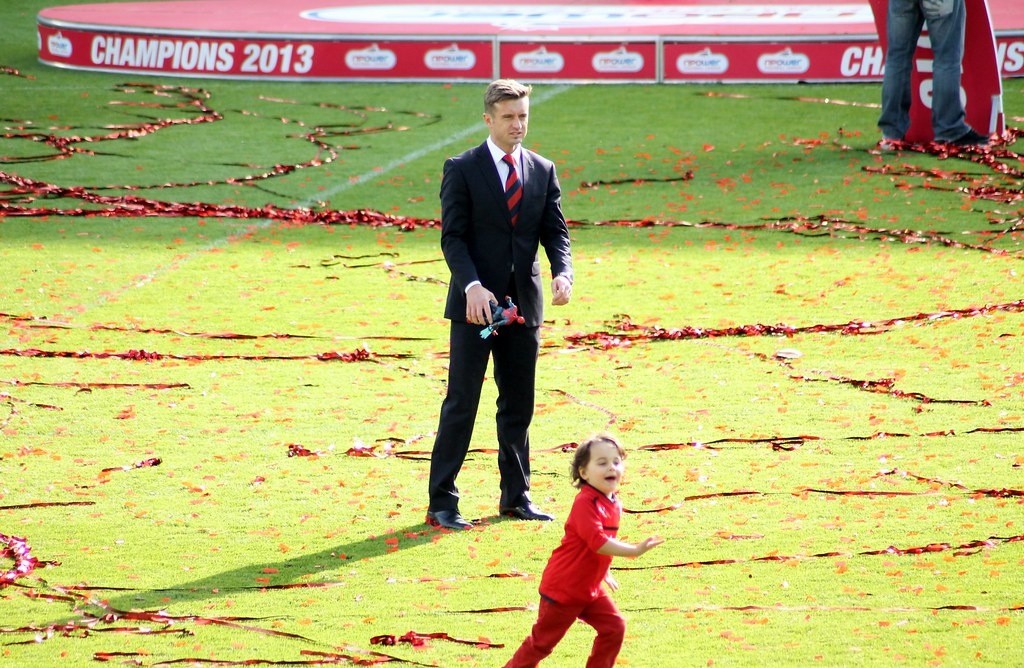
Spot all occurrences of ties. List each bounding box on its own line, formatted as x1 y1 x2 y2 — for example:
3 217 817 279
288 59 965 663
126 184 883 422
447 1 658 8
501 153 522 226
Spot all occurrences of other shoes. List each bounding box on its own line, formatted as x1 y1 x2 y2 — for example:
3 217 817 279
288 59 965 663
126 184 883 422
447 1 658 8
937 129 990 146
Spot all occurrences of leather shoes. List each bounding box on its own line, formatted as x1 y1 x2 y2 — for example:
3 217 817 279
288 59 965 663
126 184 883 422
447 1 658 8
426 509 473 529
500 501 554 520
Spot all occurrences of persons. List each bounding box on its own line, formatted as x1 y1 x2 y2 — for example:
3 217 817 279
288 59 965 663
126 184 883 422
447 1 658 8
425 77 573 528
877 1 993 148
500 437 664 668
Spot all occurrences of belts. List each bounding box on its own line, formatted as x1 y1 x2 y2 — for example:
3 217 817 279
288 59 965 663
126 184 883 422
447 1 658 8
506 264 515 272
